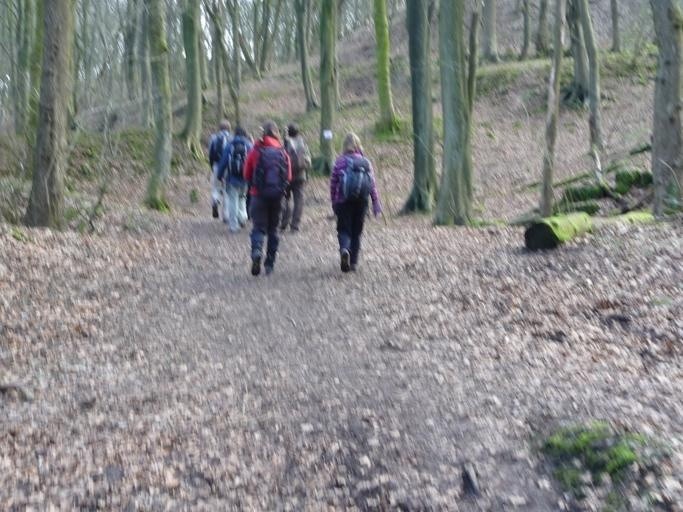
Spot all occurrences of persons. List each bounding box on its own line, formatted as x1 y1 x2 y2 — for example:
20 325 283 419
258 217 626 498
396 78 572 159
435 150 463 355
241 119 294 275
279 121 313 232
217 124 253 234
329 129 382 273
208 119 235 223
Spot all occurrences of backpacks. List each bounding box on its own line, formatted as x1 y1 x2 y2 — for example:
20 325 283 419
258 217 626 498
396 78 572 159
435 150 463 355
227 141 248 177
341 154 371 204
253 145 290 199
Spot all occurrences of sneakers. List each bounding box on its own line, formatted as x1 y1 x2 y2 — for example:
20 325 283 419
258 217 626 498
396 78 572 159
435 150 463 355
213 204 218 217
341 249 350 272
252 255 261 276
